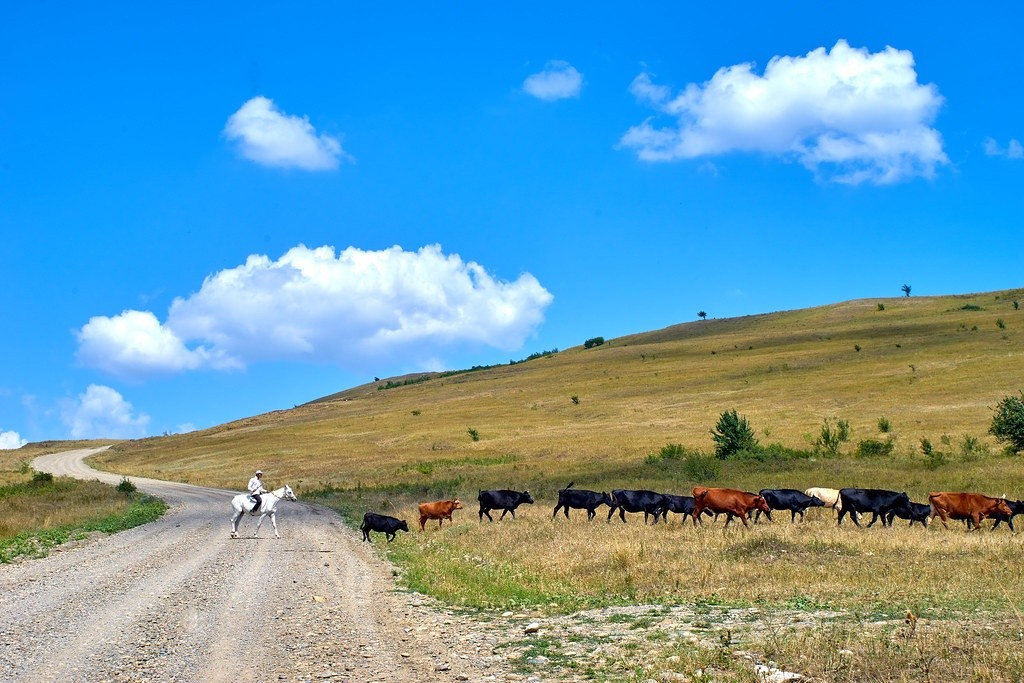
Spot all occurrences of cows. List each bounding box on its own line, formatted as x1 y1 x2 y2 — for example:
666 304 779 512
360 513 409 544
553 482 613 521
606 486 1024 532
478 489 534 522
418 498 463 531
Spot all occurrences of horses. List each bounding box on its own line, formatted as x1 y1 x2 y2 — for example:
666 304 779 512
231 484 298 539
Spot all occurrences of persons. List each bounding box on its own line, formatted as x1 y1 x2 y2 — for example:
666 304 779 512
248 470 268 516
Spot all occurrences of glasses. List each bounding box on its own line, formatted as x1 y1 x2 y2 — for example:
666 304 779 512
261 474 262 475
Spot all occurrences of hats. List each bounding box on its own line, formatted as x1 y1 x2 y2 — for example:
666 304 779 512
256 470 263 473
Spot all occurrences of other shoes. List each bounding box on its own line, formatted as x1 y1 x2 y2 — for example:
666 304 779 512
249 512 254 516
